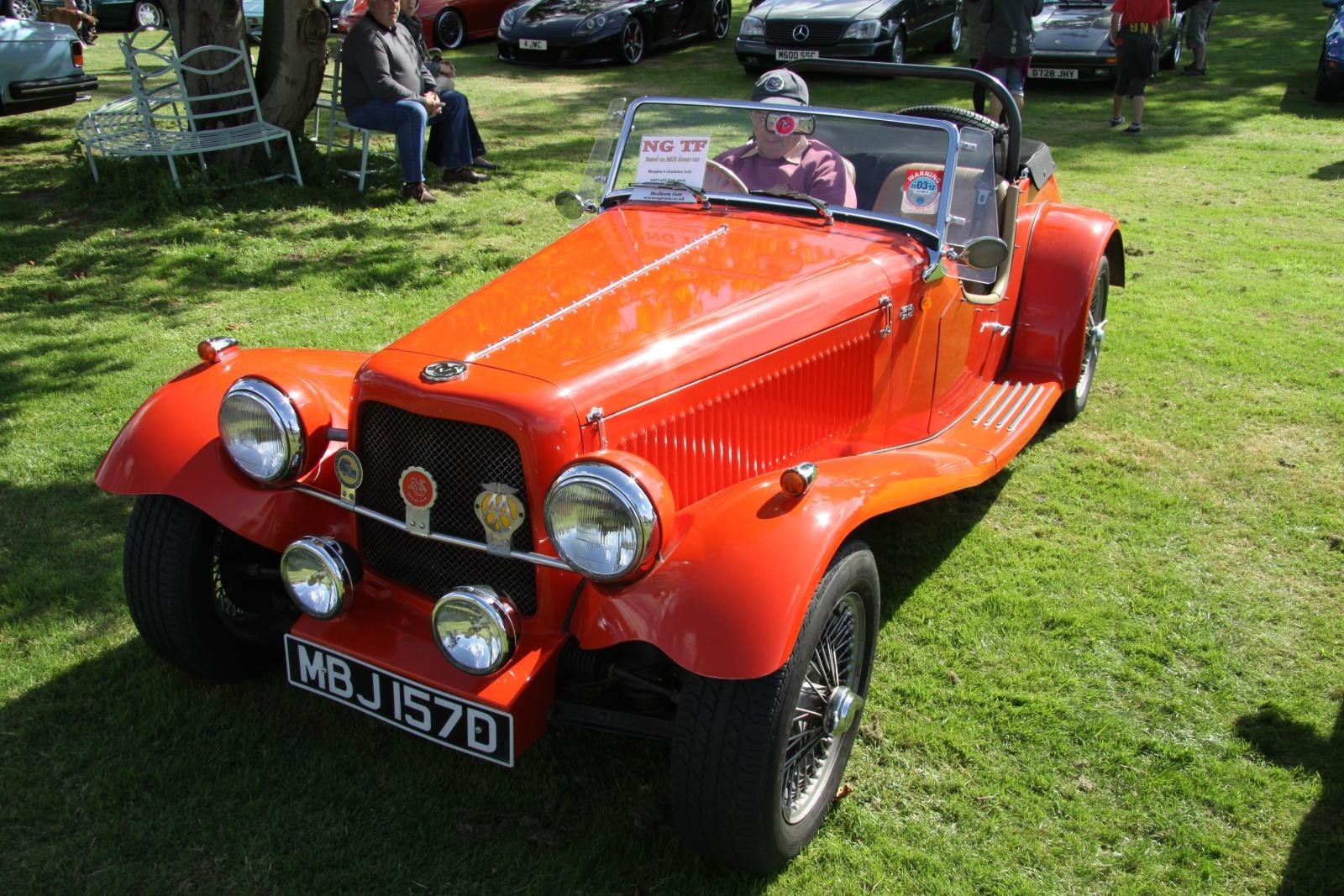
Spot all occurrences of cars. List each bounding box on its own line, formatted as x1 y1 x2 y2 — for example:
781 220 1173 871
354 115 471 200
1318 0 1344 83
0 0 168 33
95 55 1131 880
241 0 353 44
335 0 510 62
0 18 98 116
493 0 732 64
732 0 966 79
1021 0 1186 90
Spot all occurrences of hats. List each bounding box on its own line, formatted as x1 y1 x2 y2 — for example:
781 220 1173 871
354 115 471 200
751 67 809 106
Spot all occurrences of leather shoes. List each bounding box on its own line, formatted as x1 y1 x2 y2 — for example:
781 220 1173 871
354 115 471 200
402 182 438 204
442 166 491 184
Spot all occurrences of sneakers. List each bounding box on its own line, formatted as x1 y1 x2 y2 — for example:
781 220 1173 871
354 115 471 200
1110 115 1126 129
1122 126 1142 136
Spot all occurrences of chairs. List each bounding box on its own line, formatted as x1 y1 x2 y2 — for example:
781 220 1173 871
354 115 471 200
871 163 1009 294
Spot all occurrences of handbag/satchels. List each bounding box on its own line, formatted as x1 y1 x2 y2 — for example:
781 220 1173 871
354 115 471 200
420 48 455 94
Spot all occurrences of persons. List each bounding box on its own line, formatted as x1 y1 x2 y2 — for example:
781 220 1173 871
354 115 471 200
1109 0 1172 136
1170 0 1221 75
69 0 99 46
959 0 1044 125
397 0 501 171
340 0 491 202
702 68 857 209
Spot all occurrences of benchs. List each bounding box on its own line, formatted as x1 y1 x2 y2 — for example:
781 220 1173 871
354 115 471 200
60 17 403 195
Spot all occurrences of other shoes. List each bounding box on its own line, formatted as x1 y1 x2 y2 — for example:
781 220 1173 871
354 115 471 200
1179 61 1206 77
472 156 500 170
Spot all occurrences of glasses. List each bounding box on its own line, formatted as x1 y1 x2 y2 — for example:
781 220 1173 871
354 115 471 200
748 111 766 122
